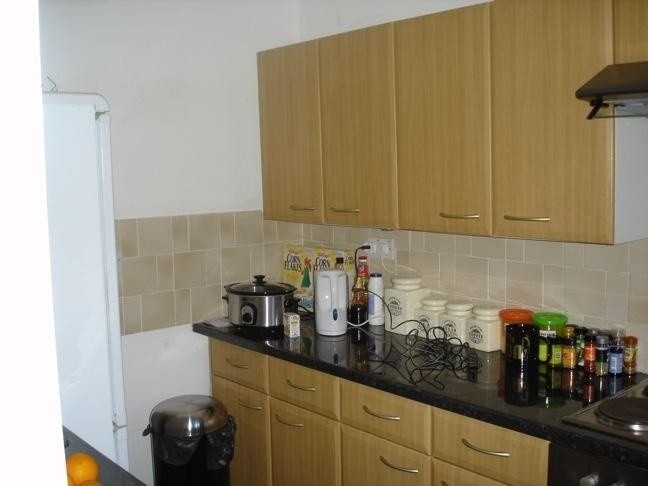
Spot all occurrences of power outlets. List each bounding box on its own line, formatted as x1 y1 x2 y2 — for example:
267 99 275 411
364 238 395 257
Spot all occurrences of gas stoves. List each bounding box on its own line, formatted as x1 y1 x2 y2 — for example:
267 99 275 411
561 378 648 446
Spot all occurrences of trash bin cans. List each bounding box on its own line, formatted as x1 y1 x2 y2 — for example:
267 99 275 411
142 394 238 486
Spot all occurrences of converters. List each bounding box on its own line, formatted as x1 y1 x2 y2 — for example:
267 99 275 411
467 348 478 370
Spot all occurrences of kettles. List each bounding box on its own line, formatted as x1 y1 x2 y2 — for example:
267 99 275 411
313 269 348 337
316 335 347 368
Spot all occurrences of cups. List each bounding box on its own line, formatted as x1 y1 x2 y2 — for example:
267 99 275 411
503 370 552 407
505 323 553 369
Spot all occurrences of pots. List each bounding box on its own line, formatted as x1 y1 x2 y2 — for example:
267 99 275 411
221 275 301 340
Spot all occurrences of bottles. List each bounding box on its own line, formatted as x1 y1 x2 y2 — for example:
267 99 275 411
538 367 563 402
351 256 370 310
562 327 638 376
350 346 367 377
367 273 385 325
369 326 386 372
351 289 366 346
532 312 568 367
500 310 534 354
562 368 634 409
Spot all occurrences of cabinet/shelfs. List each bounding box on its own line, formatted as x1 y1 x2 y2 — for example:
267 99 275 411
396 0 615 261
256 19 399 232
614 1 648 67
208 337 342 485
341 378 550 485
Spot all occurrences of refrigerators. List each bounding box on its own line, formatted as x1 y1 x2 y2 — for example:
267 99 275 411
43 92 130 472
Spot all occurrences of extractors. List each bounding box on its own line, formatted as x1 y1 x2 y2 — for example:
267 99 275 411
575 61 648 121
